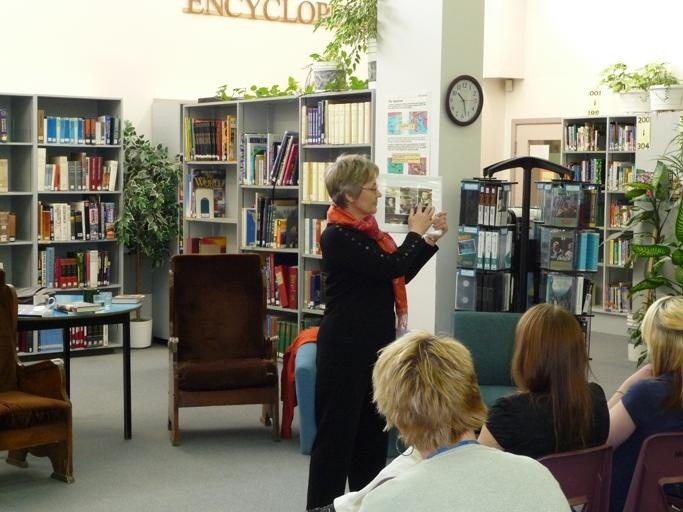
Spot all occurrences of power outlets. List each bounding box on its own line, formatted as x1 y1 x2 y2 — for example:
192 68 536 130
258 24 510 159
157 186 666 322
560 110 683 315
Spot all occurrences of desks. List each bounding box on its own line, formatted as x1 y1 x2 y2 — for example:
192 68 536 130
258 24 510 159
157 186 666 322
17 296 142 442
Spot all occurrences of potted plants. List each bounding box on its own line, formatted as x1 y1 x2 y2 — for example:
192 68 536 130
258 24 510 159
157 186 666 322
301 0 378 95
602 58 647 112
117 121 180 352
596 159 682 363
646 60 682 111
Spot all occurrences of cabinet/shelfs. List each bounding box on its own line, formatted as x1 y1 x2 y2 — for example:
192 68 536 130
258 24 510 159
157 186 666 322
0 93 32 290
182 96 297 352
299 91 374 313
34 100 125 353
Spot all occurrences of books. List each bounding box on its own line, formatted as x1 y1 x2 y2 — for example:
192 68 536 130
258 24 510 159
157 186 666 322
240 131 299 185
304 217 327 256
191 236 227 254
448 113 639 315
185 168 226 218
1 102 144 353
301 100 372 146
241 192 298 248
262 253 298 309
301 315 321 331
184 114 237 162
304 269 327 309
264 313 298 358
302 161 337 202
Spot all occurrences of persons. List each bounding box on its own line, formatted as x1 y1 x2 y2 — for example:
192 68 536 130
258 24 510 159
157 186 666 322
302 152 449 510
333 329 574 509
458 303 613 459
605 292 682 511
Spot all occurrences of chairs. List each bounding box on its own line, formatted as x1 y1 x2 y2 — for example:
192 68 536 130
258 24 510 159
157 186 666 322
294 326 415 459
164 251 281 445
535 443 621 511
0 268 75 483
626 431 682 512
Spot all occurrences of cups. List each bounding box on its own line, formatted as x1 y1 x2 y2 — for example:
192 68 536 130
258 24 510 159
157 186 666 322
92 295 105 313
32 294 55 315
99 291 112 310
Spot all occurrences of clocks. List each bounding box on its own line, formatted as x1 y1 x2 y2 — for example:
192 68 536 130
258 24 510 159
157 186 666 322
443 74 484 126
